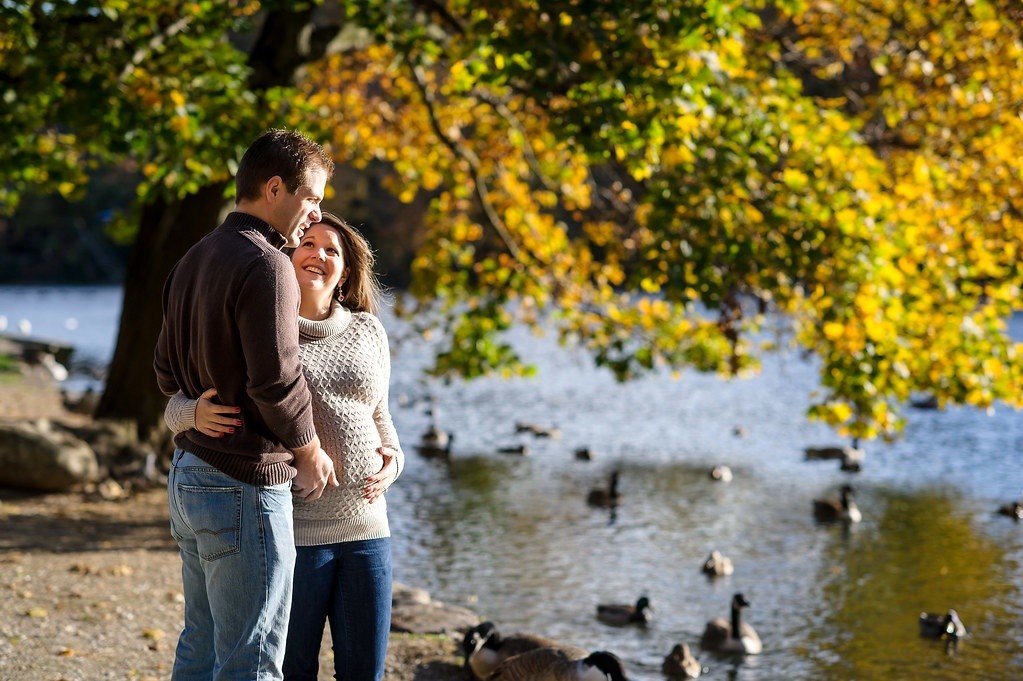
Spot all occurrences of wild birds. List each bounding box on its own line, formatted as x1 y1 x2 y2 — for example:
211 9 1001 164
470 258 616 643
660 643 702 680
998 500 1023 521
812 482 863 528
702 549 735 578
906 390 941 413
700 591 764 656
496 420 563 455
597 593 656 626
587 466 623 508
801 436 866 472
916 605 968 643
709 462 733 483
409 407 455 466
463 620 627 681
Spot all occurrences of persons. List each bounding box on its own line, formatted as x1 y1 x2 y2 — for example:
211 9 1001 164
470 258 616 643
164 212 405 681
155 127 341 681
